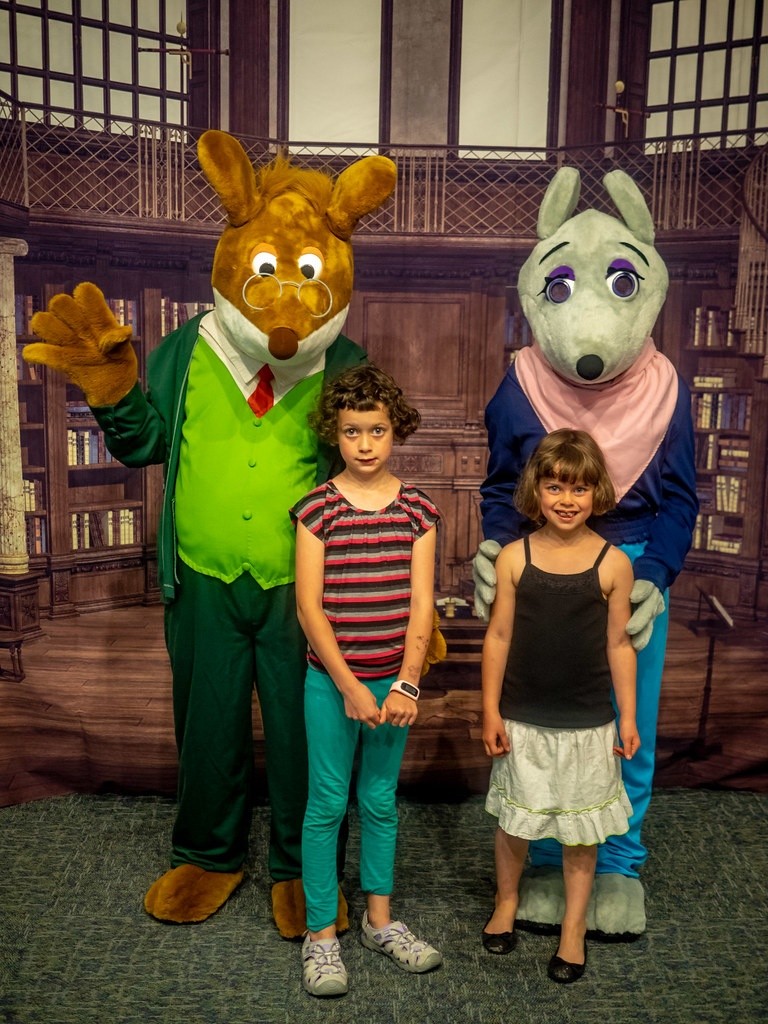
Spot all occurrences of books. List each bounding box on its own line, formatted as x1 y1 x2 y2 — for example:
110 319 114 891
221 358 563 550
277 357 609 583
684 301 750 553
14 296 217 557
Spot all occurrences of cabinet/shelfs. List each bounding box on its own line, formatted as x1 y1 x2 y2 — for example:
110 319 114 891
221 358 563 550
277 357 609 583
15 282 216 620
664 278 768 622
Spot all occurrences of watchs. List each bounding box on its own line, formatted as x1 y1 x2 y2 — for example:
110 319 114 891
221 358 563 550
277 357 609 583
390 680 420 703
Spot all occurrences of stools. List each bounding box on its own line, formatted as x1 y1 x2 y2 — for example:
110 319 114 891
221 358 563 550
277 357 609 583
0 630 26 683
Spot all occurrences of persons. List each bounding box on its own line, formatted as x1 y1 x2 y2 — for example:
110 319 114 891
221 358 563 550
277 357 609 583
481 429 640 983
287 367 446 996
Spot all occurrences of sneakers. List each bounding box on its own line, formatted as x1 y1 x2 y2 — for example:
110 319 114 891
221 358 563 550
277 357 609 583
300 931 349 995
361 909 443 972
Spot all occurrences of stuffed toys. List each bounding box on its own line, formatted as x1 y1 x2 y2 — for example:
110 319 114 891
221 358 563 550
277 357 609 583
472 165 699 938
21 129 444 935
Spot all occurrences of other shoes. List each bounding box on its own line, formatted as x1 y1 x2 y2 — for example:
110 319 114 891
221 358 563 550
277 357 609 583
547 939 588 985
482 906 518 955
